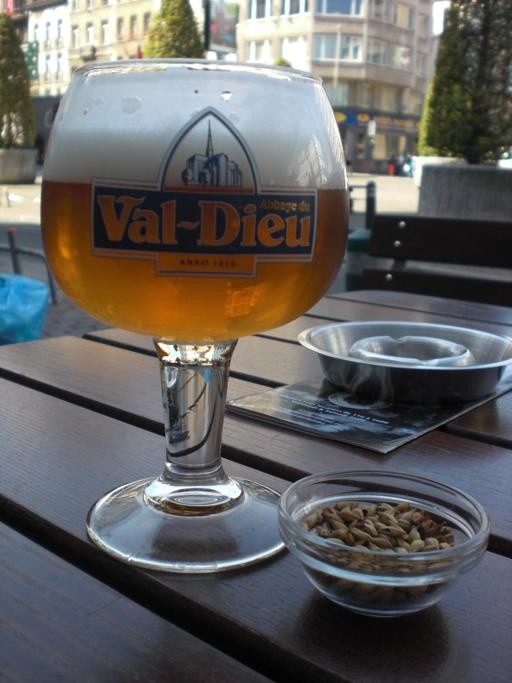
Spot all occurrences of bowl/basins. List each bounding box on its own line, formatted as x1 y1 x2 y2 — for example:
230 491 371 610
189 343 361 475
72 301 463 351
276 468 493 621
297 318 512 406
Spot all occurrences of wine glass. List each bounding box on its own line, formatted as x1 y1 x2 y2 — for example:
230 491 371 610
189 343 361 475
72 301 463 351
37 56 353 577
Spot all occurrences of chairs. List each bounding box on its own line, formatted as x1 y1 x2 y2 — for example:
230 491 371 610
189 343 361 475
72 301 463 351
364 212 511 306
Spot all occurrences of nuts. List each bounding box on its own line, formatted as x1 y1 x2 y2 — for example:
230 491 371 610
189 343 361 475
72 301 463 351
302 498 455 609
